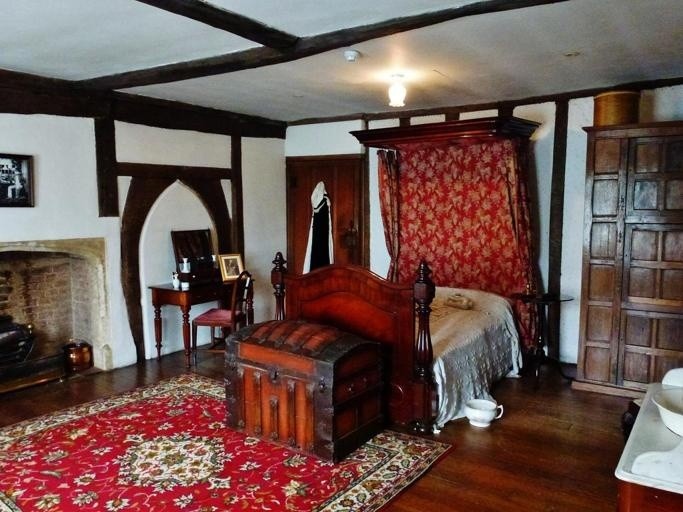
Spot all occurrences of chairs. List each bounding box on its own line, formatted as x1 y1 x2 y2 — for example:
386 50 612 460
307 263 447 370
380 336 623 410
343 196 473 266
190 269 251 366
662 365 683 389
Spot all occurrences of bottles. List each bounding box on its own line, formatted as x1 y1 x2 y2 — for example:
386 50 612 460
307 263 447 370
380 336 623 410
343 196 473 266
180 257 190 288
171 273 179 288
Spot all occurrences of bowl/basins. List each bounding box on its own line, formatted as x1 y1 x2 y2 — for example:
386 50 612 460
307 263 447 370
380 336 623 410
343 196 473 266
650 385 683 437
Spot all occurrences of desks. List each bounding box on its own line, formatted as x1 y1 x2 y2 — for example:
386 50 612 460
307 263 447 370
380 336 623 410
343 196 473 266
611 382 682 511
512 289 576 391
147 277 254 371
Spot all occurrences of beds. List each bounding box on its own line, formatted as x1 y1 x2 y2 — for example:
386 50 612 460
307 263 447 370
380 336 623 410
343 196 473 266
269 113 540 432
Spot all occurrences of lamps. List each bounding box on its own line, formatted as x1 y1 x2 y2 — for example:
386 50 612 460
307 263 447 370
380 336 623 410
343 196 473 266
385 74 406 107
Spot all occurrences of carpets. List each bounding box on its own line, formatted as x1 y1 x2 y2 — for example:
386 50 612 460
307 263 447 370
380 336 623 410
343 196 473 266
1 370 453 512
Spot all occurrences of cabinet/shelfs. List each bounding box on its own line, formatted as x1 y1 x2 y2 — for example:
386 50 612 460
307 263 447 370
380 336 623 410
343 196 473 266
571 119 683 397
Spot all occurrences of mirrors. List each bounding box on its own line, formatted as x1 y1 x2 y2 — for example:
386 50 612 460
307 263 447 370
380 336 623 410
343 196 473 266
170 228 220 285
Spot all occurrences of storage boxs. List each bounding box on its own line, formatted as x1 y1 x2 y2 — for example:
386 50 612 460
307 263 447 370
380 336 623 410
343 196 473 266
222 317 394 464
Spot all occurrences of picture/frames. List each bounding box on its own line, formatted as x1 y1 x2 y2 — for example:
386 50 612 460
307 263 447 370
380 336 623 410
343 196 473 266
0 151 35 209
217 254 244 282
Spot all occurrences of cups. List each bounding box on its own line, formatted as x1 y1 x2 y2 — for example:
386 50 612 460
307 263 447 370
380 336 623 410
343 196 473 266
61 342 90 372
464 398 503 427
